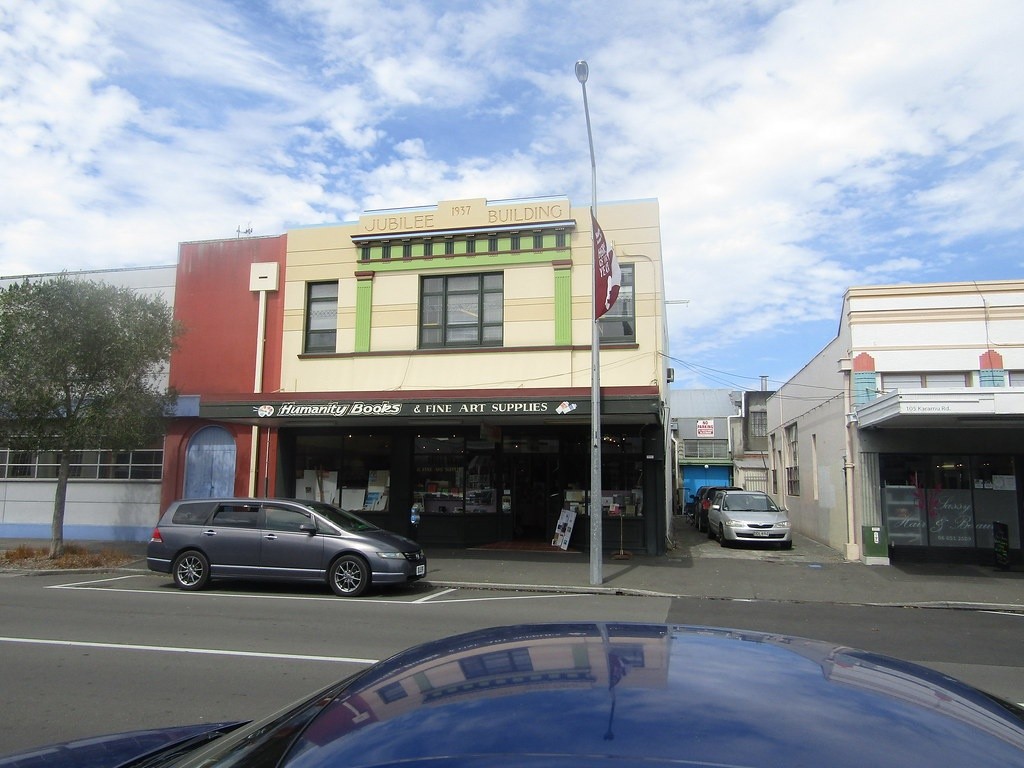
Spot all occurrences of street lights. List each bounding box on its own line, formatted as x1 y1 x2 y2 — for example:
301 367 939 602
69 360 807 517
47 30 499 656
574 57 605 585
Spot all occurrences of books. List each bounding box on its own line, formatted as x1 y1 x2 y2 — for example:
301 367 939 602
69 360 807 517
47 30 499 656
428 484 458 498
566 491 591 515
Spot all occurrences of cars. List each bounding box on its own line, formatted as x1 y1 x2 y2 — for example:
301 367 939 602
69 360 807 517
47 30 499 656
689 486 744 532
145 498 428 597
707 491 793 551
1 620 1024 768
